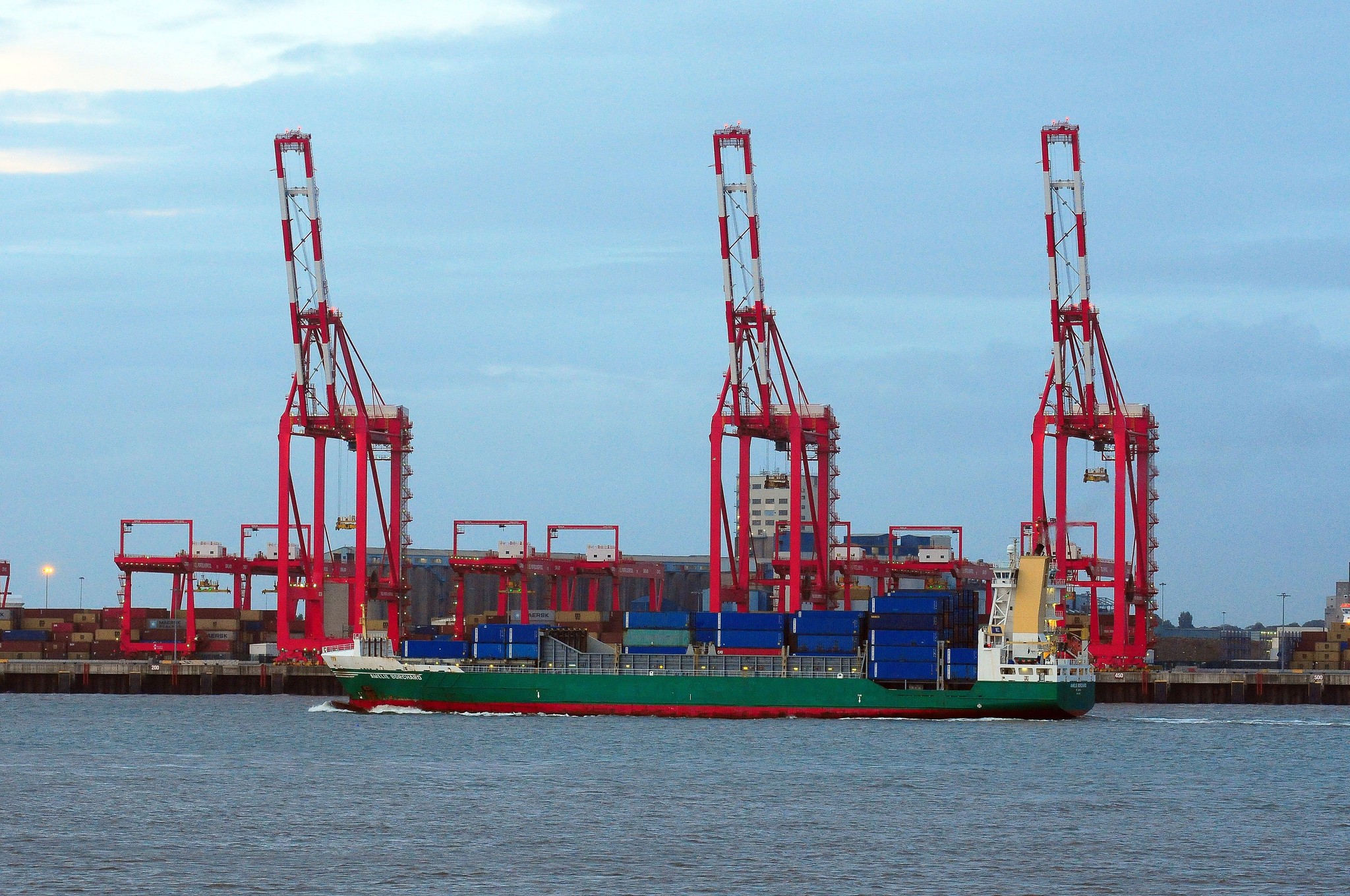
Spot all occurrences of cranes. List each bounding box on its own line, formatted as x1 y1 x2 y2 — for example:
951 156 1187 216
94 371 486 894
274 132 412 662
1029 117 1159 671
710 128 839 657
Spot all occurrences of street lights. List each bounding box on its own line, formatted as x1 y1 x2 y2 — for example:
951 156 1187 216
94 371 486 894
1159 583 1166 619
44 568 52 609
1276 593 1291 670
1222 612 1226 630
79 577 84 609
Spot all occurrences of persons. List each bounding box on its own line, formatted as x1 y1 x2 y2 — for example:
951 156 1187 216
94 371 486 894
1162 659 1176 671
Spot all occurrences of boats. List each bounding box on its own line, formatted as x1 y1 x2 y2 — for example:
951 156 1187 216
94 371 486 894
321 538 1095 719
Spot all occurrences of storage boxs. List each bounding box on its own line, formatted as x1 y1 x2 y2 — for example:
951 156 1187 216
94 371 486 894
1065 613 1271 662
366 610 623 659
624 590 978 680
0 607 306 660
1289 622 1350 670
